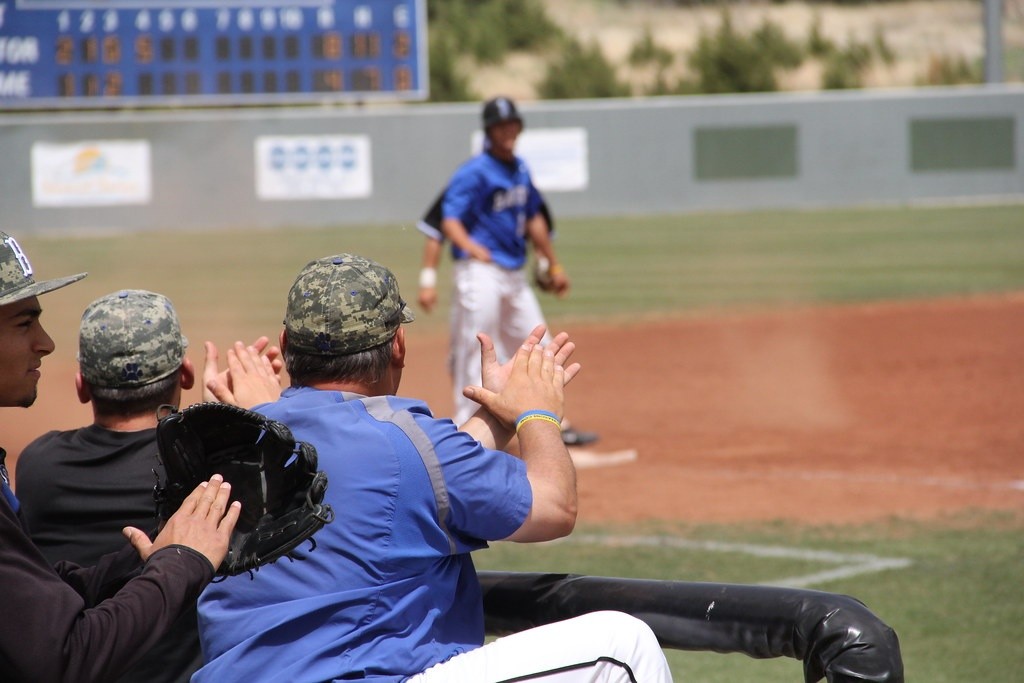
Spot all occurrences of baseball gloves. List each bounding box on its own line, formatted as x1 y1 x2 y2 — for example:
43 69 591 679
151 401 336 576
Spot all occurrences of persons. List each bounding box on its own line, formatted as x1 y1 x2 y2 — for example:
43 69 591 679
0 228 242 683
190 253 673 683
15 287 283 568
414 95 599 446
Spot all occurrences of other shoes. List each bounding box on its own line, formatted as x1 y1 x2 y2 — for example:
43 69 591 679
561 426 602 445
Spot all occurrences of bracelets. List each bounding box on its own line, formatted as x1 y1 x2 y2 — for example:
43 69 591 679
418 267 437 288
514 409 562 439
549 264 563 276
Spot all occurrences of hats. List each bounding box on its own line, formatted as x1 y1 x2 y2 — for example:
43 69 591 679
76 289 189 390
283 253 415 358
0 230 88 307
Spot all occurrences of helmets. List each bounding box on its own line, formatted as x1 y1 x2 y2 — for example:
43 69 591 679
482 97 523 138
